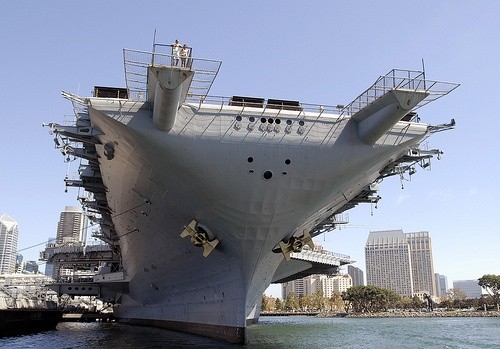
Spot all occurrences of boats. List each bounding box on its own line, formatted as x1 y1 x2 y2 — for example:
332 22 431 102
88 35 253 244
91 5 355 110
0 305 64 336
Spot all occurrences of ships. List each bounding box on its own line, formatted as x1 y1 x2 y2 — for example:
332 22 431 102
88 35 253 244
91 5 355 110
41 28 460 343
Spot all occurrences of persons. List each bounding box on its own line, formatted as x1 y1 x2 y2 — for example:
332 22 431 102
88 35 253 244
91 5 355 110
172 39 182 66
180 44 189 67
